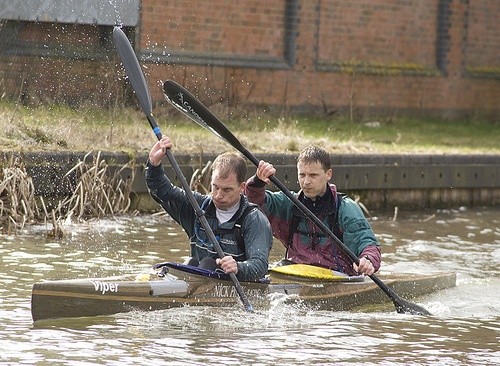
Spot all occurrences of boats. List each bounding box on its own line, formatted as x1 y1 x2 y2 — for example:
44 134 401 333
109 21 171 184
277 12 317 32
31 270 459 325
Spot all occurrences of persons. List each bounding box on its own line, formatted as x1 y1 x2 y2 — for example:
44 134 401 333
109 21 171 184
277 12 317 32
245 144 382 277
145 135 274 282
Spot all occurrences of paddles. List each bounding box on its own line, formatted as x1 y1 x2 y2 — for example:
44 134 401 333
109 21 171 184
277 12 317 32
111 26 253 314
163 79 434 320
